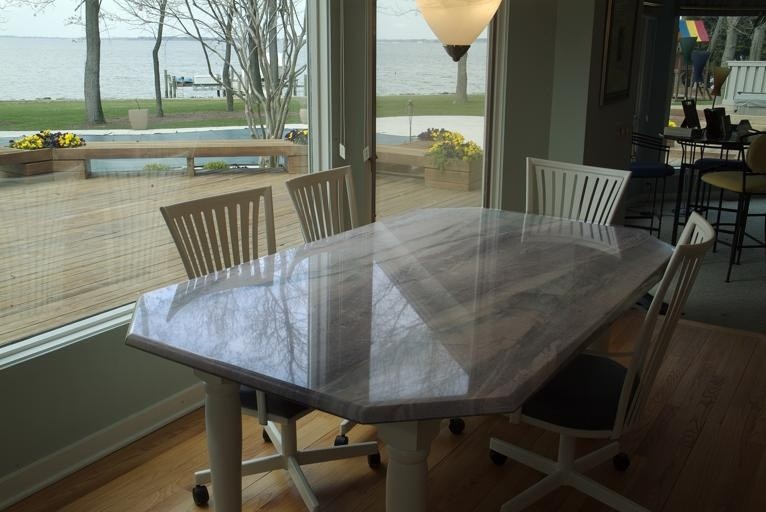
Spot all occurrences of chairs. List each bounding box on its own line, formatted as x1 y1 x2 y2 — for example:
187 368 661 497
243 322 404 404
630 100 766 283
524 158 634 225
285 163 466 449
159 186 382 511
487 211 716 509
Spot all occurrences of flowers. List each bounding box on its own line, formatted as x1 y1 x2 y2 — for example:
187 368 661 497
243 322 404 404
285 129 309 145
424 138 486 172
416 128 464 144
7 129 86 150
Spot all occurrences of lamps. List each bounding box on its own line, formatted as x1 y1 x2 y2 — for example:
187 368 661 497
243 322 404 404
417 0 503 64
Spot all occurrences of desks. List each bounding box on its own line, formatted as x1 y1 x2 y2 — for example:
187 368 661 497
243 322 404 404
126 207 676 512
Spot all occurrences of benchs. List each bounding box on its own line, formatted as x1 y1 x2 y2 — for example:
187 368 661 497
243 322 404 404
85 138 289 176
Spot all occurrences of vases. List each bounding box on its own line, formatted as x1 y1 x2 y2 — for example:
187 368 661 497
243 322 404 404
0 146 91 182
424 154 482 192
281 149 310 173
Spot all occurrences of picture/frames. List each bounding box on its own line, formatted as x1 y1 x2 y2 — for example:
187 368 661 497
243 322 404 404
600 1 640 105
